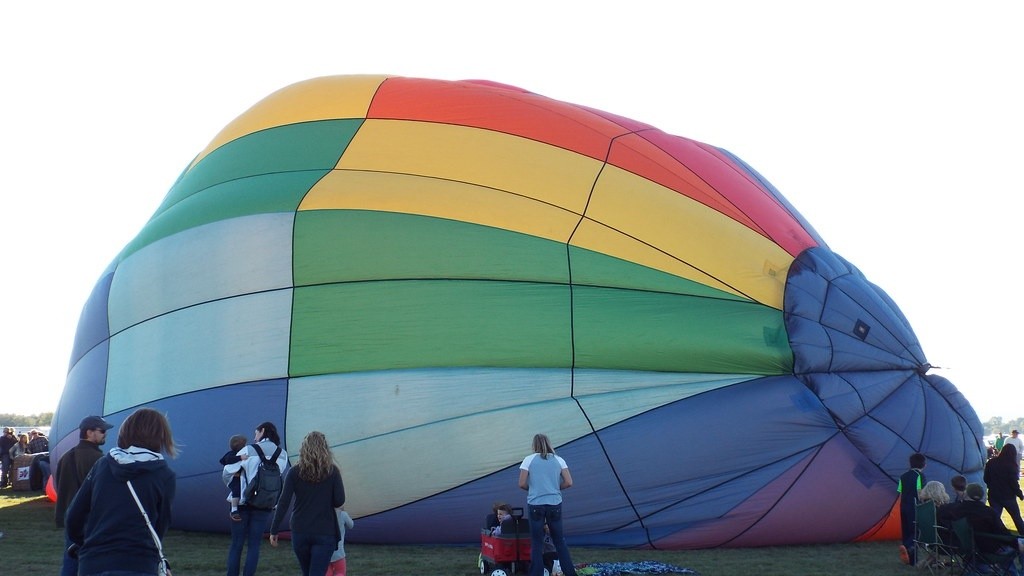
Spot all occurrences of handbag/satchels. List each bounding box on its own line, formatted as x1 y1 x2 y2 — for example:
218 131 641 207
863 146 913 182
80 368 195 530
67 543 79 559
157 557 171 576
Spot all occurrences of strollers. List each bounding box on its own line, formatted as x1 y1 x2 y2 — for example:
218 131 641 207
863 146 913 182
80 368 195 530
480 506 551 576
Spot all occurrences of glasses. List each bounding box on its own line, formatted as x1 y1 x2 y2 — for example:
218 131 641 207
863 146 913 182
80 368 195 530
93 428 107 435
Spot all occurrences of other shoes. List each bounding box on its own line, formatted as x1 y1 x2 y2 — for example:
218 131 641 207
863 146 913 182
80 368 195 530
230 510 242 522
899 545 909 564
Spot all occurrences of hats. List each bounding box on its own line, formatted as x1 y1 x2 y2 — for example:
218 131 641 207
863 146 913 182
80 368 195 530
79 415 114 431
1012 430 1020 434
967 483 985 501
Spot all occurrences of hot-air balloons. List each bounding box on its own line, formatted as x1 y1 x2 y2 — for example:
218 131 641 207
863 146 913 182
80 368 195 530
8 74 989 551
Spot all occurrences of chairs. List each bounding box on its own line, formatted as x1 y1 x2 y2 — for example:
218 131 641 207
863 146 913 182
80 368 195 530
911 496 969 576
948 517 1022 576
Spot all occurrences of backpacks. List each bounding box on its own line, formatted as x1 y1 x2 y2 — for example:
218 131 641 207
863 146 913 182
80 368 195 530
242 444 284 514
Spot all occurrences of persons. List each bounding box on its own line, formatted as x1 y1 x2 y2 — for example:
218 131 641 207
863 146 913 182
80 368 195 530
66 409 186 576
486 433 579 576
219 422 354 576
988 430 1024 479
984 443 1024 536
896 453 1024 576
0 428 50 494
55 416 114 576
